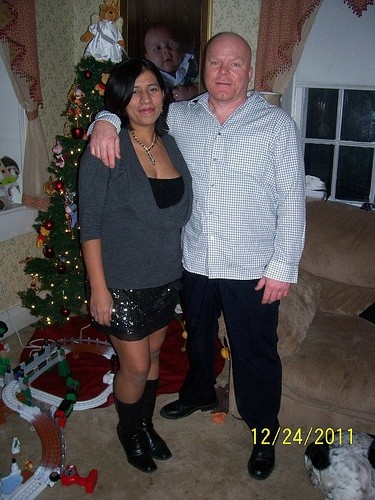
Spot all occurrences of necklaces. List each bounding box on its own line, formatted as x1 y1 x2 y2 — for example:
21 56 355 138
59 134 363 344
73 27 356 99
131 130 157 165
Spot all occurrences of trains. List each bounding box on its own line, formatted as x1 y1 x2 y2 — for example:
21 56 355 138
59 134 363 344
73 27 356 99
54 378 79 420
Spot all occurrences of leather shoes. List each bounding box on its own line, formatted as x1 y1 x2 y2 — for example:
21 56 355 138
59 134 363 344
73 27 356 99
160 398 219 419
248 425 280 480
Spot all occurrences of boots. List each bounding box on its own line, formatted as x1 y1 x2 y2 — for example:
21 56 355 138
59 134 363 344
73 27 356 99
141 377 172 459
112 391 158 473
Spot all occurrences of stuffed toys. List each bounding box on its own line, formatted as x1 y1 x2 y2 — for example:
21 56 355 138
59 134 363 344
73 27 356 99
80 2 125 63
65 202 78 228
52 144 68 168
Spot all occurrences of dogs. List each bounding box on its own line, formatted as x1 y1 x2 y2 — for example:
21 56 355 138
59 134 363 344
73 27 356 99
305 429 375 500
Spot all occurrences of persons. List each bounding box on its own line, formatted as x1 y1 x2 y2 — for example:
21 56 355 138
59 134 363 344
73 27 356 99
79 58 192 473
143 22 199 102
87 31 306 480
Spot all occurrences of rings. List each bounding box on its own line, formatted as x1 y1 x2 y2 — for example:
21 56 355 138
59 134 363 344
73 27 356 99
176 94 177 97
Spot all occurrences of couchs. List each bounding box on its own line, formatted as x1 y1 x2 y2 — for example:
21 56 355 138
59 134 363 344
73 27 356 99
215 195 375 438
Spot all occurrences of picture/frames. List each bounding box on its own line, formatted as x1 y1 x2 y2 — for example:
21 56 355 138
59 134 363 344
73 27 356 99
120 0 213 103
